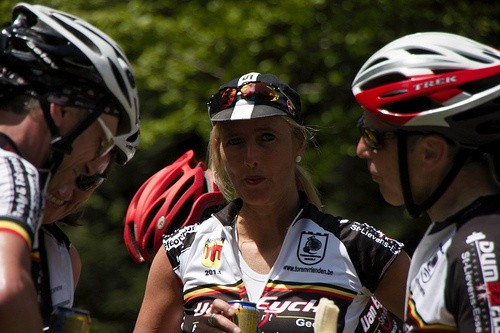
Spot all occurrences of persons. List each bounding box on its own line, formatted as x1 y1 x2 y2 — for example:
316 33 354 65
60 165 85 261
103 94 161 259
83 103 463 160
0 3 140 332
351 32 500 333
132 73 412 333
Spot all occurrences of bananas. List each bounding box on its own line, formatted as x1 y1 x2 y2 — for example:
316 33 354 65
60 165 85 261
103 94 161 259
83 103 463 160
312 297 340 333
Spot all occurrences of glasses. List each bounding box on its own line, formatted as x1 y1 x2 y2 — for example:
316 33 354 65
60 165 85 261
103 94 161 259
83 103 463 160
207 81 301 126
72 168 105 192
356 114 457 150
89 109 120 162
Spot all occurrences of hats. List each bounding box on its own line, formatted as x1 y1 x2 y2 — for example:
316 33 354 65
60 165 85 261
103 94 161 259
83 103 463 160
208 71 301 121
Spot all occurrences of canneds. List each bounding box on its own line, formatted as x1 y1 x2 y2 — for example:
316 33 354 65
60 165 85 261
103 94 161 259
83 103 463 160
47 304 92 333
223 300 257 333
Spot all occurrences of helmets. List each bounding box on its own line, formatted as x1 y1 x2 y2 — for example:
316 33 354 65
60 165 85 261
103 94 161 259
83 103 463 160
0 2 140 166
351 31 500 151
123 149 229 264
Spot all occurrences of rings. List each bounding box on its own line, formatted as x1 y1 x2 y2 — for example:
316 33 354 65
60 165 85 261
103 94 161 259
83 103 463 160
208 313 216 328
